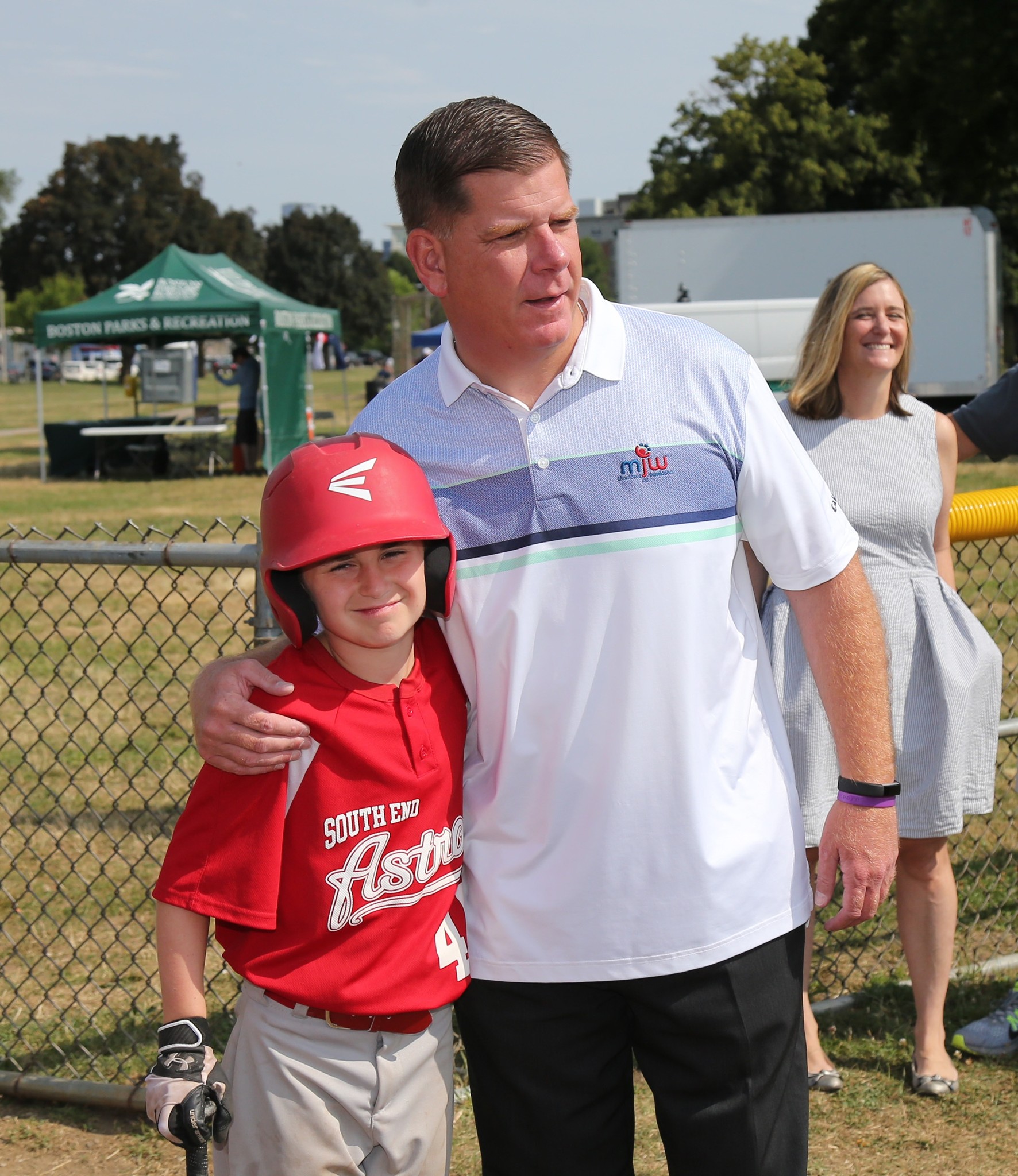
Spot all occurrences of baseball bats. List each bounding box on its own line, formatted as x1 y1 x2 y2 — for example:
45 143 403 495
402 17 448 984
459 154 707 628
183 1100 217 1176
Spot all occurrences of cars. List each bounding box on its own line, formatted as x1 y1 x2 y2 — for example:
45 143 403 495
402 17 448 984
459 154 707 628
9 342 125 386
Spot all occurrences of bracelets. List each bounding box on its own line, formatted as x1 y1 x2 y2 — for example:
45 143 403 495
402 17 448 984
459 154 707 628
837 776 901 809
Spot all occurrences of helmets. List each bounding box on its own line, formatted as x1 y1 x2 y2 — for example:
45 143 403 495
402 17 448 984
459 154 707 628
259 432 456 650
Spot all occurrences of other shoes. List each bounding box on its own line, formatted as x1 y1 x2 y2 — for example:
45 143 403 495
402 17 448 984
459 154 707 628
807 1049 846 1091
910 1049 960 1096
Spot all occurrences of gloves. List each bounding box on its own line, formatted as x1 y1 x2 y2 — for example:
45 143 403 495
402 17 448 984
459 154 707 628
147 1017 232 1152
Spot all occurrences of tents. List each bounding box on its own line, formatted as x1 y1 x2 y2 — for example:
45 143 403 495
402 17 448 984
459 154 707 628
34 243 350 484
411 320 448 367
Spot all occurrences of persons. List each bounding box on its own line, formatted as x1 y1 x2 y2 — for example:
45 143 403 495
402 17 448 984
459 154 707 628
189 99 900 1176
420 346 433 360
211 345 260 472
945 363 1018 464
17 345 60 386
141 433 469 1176
674 282 691 303
742 259 1003 1094
374 355 395 396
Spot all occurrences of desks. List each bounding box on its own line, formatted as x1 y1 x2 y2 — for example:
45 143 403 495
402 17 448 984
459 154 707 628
44 413 186 479
82 424 229 479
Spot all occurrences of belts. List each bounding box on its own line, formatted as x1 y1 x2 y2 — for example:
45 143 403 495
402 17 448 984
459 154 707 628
264 990 433 1034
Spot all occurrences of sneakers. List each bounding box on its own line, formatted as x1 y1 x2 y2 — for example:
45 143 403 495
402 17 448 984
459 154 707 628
949 992 1018 1055
